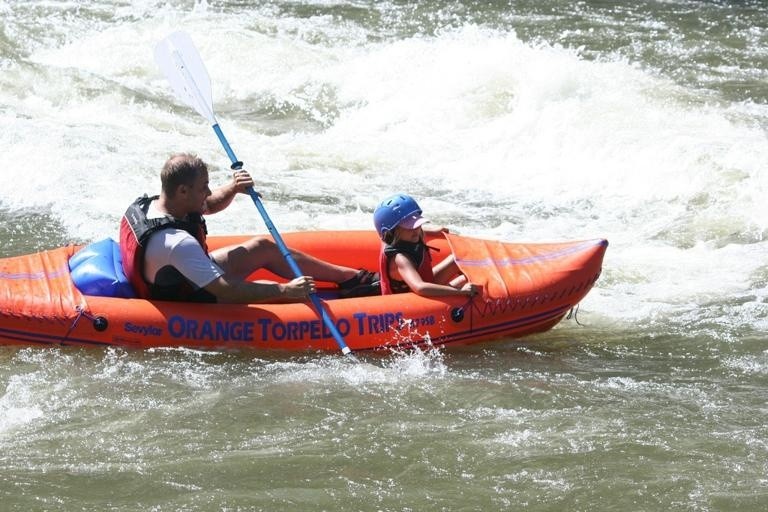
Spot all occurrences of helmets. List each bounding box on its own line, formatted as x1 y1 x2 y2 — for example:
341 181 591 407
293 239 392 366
373 194 422 240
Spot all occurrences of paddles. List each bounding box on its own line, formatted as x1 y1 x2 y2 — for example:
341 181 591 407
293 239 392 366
151 30 360 364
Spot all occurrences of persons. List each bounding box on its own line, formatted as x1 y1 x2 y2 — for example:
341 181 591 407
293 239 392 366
372 193 479 298
119 153 381 303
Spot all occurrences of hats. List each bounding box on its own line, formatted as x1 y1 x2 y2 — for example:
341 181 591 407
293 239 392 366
399 213 430 229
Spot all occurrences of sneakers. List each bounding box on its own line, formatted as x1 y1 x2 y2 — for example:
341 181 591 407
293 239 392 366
338 268 380 297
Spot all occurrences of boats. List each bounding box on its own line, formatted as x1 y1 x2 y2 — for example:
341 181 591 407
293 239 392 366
0 226 612 358
